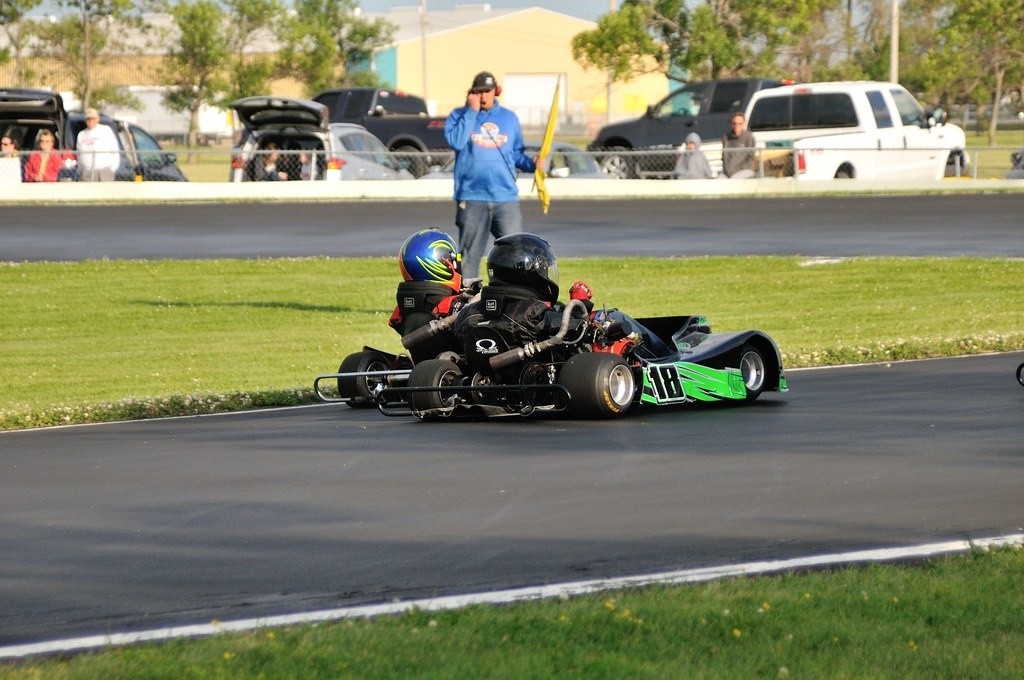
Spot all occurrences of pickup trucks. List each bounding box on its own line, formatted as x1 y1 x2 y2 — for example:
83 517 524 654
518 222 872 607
311 88 456 179
673 80 971 181
585 78 805 180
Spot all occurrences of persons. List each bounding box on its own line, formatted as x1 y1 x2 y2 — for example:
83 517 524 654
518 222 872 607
0 128 76 182
689 94 702 115
388 228 483 335
717 113 756 179
674 132 713 179
253 142 322 181
454 233 676 361
444 71 547 279
76 107 120 182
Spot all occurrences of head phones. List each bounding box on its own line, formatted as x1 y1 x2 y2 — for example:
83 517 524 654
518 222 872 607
495 83 501 97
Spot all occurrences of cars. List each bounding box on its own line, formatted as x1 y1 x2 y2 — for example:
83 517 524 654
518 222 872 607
417 140 615 179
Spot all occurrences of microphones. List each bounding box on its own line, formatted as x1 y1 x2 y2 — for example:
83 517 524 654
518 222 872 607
480 102 486 105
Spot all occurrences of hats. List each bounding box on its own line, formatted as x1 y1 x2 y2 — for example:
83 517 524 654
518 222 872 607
84 108 98 119
471 71 496 92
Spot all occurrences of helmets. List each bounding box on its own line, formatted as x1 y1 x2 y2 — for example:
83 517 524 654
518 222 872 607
487 232 560 301
399 227 464 293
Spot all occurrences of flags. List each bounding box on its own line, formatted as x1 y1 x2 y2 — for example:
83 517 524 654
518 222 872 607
532 81 561 213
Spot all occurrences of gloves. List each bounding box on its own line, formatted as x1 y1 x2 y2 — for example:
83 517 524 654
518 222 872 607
570 281 594 303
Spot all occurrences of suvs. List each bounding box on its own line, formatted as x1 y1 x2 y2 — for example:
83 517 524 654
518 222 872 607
0 88 189 183
229 96 415 182
